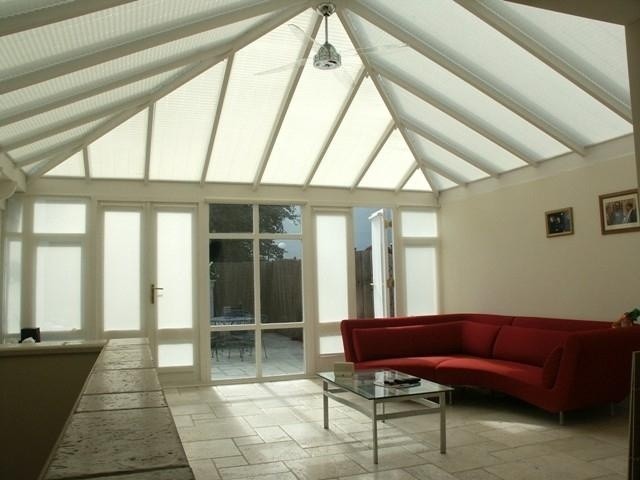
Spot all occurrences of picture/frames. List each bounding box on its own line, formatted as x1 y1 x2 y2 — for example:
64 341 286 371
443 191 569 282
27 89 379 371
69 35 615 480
545 206 575 238
597 188 639 236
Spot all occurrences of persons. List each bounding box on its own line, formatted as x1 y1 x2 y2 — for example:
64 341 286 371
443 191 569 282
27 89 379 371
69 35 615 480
606 202 636 225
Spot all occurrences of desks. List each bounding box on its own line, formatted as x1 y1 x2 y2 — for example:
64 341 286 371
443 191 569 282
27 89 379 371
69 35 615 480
211 315 255 359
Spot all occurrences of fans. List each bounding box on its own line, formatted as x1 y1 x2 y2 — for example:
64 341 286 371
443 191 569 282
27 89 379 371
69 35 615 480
255 3 408 79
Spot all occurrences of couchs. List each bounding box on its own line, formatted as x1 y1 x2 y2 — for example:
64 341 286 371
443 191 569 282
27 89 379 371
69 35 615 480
341 313 639 425
